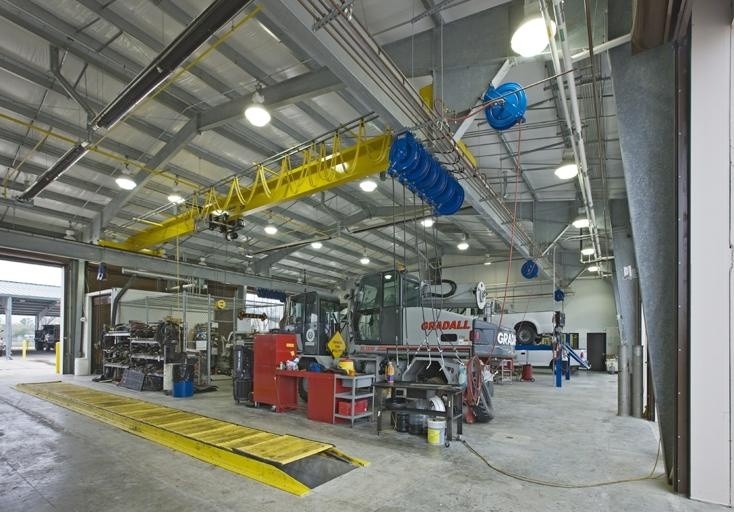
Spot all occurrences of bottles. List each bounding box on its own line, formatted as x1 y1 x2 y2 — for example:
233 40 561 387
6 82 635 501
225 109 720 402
386 361 394 385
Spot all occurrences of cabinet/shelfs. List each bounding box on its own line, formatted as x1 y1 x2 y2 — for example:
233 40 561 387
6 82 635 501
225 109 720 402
251 333 295 409
102 330 178 395
331 373 375 428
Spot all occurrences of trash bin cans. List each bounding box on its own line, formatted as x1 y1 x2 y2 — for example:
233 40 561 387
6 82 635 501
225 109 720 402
172 364 195 397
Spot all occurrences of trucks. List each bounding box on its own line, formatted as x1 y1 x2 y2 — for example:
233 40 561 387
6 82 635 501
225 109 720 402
278 268 517 409
37 324 60 352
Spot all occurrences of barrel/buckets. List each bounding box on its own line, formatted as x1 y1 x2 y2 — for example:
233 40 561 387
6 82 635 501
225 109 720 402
427 418 447 445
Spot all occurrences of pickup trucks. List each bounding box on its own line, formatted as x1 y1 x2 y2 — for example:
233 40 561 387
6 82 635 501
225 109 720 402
466 300 557 347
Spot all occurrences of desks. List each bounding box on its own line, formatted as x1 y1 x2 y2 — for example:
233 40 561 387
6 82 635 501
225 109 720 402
373 381 465 447
274 368 353 424
